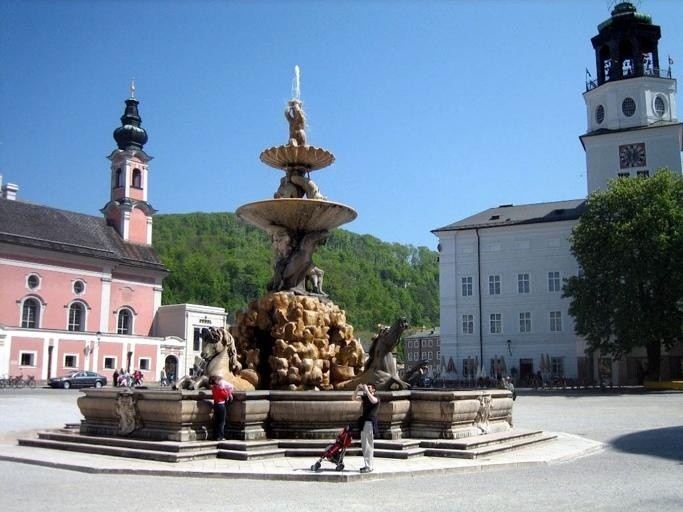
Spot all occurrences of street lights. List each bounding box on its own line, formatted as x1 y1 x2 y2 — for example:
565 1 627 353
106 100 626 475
127 351 132 371
48 345 53 378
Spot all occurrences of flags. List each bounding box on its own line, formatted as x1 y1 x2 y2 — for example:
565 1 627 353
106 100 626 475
586 68 591 76
669 58 673 64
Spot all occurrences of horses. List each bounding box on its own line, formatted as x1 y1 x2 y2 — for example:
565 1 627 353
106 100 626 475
335 316 411 391
173 326 257 391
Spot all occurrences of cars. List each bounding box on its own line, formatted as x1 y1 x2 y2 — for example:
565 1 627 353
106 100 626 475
47 371 106 390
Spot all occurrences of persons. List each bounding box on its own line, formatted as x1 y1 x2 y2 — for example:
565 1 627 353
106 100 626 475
114 368 143 387
218 377 234 403
160 367 167 386
209 376 229 441
285 100 305 146
169 371 174 385
351 383 380 473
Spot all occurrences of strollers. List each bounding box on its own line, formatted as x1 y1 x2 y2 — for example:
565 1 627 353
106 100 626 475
311 424 354 471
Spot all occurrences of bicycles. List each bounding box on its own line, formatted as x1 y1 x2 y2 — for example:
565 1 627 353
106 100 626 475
0 374 35 390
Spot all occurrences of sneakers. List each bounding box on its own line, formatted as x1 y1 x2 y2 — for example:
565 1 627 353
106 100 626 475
360 467 373 473
